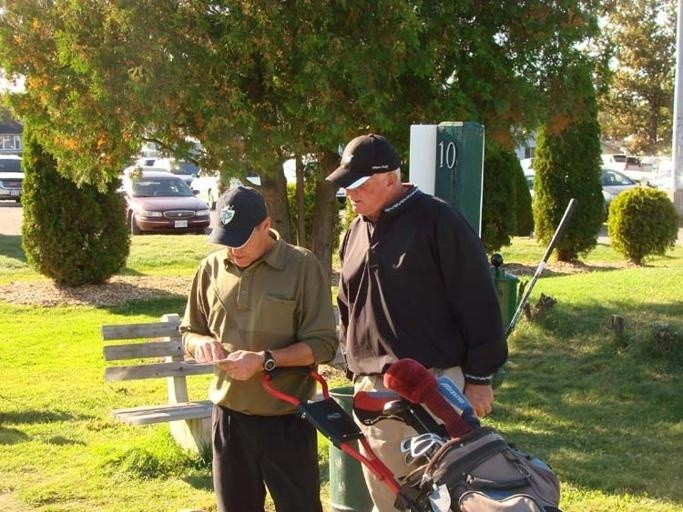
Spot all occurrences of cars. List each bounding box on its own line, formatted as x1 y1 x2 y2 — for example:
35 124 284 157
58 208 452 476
520 150 674 218
100 135 348 237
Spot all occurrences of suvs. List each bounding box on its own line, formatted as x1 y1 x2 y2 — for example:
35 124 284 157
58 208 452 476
0 154 25 206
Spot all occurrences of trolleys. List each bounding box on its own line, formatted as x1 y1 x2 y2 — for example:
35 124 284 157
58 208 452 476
259 363 416 511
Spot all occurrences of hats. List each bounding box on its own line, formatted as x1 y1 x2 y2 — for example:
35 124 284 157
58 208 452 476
207 185 268 247
325 133 399 187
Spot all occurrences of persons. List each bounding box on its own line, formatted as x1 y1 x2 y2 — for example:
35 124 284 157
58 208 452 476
179 183 338 512
323 134 508 512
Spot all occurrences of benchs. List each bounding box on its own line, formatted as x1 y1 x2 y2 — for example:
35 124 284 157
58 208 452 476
100 306 363 459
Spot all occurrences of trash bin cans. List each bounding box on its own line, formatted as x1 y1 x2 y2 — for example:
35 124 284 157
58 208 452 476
488 254 521 332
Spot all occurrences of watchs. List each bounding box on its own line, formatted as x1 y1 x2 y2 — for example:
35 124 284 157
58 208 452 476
262 347 276 375
